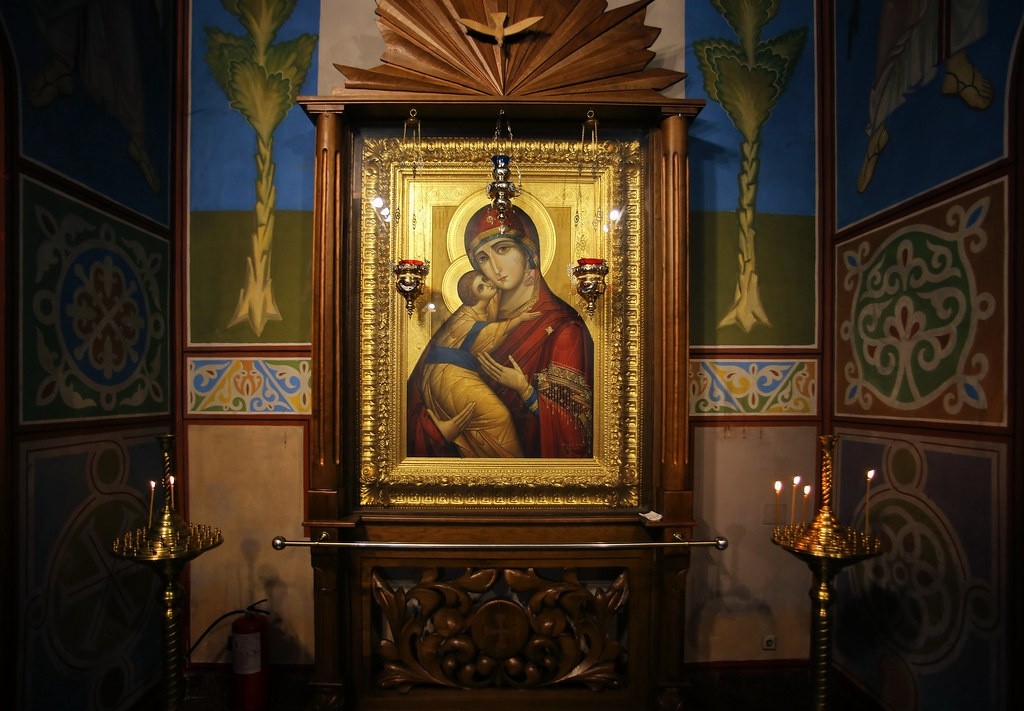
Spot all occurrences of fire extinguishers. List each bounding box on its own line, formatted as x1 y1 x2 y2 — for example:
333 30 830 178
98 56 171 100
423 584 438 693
225 599 272 711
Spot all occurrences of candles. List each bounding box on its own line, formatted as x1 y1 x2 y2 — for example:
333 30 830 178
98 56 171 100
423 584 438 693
147 476 178 531
775 469 875 535
